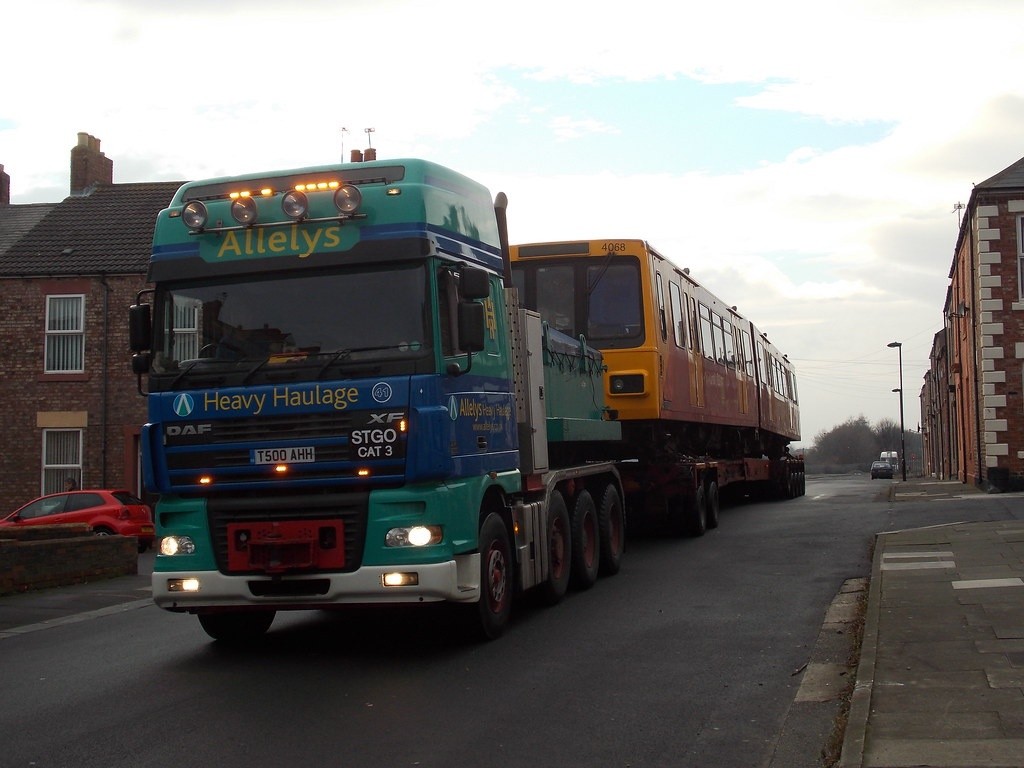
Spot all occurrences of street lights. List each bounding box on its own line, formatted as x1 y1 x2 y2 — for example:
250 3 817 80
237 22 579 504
886 341 908 480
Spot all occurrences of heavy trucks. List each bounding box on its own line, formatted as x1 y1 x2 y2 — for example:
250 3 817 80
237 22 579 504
128 146 807 647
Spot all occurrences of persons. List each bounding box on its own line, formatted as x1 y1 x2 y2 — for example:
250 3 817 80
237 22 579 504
64 479 78 492
215 311 298 354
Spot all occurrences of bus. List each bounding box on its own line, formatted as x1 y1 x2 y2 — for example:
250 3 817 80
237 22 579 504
500 237 805 444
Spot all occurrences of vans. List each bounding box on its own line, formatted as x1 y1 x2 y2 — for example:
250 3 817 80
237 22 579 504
880 451 898 473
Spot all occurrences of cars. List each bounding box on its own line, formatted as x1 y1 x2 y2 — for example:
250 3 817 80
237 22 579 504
871 461 893 479
0 488 154 556
